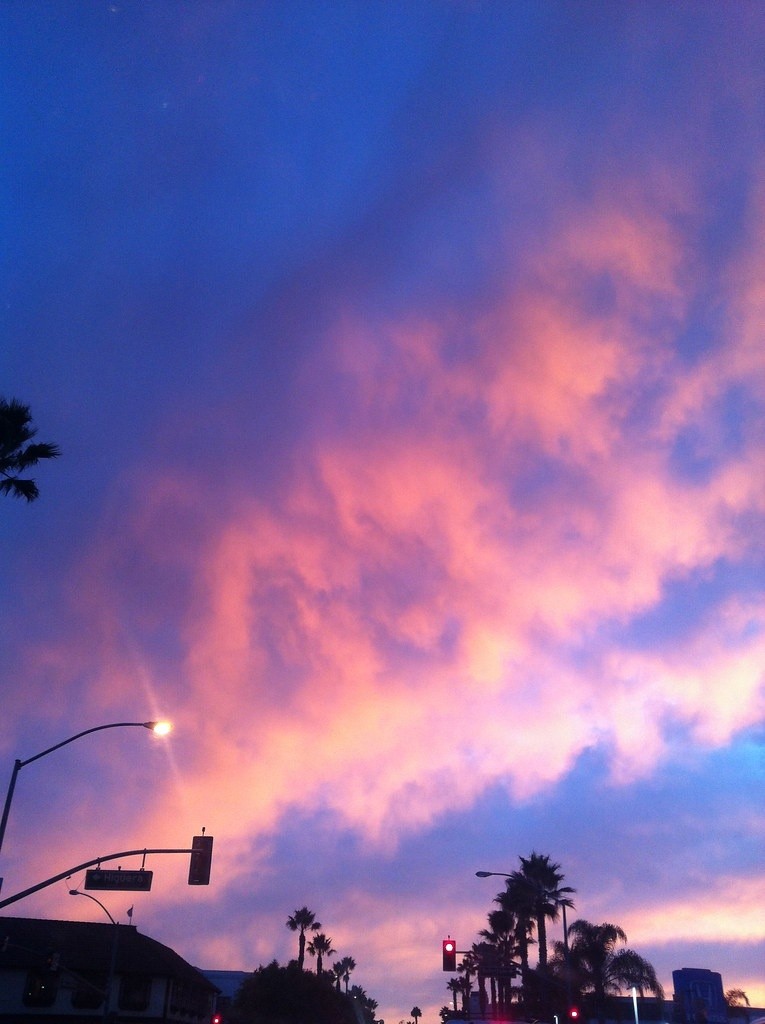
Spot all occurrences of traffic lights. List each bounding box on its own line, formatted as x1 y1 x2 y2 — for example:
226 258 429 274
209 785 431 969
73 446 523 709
443 937 457 972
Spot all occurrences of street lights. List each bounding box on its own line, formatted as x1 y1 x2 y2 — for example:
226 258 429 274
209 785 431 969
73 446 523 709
475 870 574 1003
0 720 170 851
67 888 123 1024
